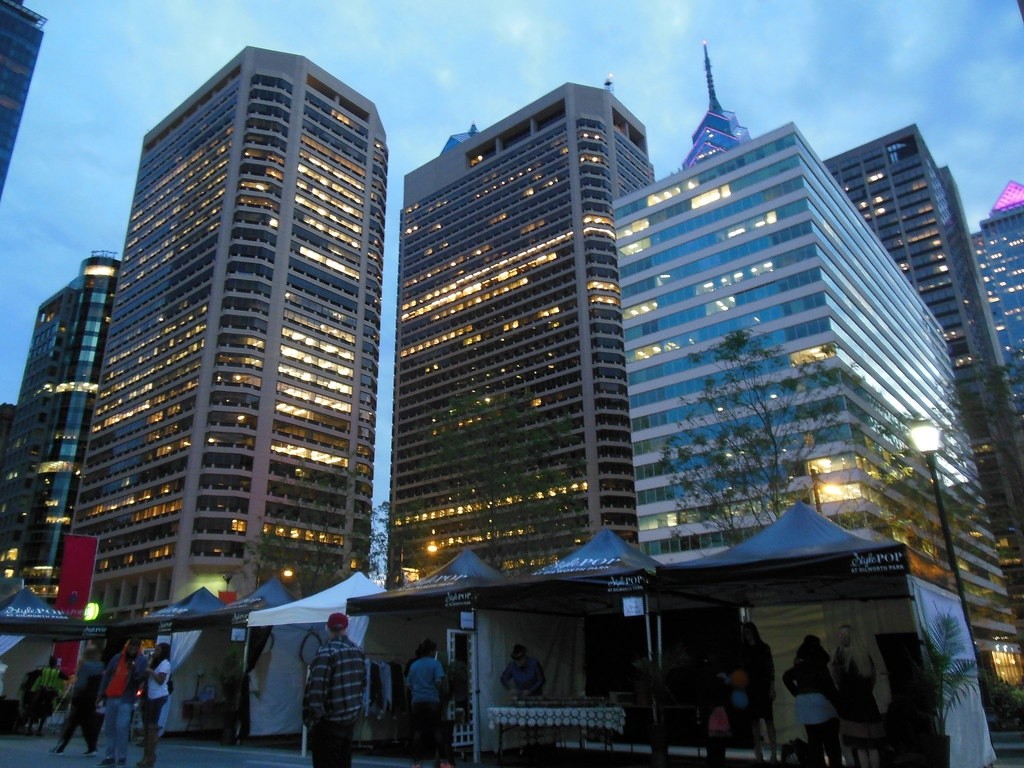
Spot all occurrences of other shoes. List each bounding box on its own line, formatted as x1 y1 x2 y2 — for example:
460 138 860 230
440 762 455 768
117 757 127 767
413 765 422 768
83 749 97 756
97 758 115 767
48 750 63 755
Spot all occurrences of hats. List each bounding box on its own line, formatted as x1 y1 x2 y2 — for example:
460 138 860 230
511 644 527 660
327 613 349 631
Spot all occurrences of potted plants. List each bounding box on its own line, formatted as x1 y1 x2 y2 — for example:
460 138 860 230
910 613 981 768
210 646 261 746
433 661 473 768
631 642 692 768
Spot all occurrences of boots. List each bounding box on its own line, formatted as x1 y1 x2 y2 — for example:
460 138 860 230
136 725 160 767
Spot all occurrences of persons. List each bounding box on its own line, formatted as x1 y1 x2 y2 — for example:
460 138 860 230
500 645 545 702
303 613 366 768
404 639 454 768
741 621 888 768
666 636 733 768
13 637 171 767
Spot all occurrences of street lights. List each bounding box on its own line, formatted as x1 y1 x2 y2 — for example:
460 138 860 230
903 418 995 711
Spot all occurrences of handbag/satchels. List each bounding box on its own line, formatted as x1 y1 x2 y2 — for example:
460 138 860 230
167 680 174 694
708 705 732 738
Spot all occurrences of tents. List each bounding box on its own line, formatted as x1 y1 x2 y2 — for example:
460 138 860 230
0 525 666 768
656 502 997 768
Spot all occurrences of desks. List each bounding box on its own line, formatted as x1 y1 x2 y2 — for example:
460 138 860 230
488 707 627 768
181 702 230 738
